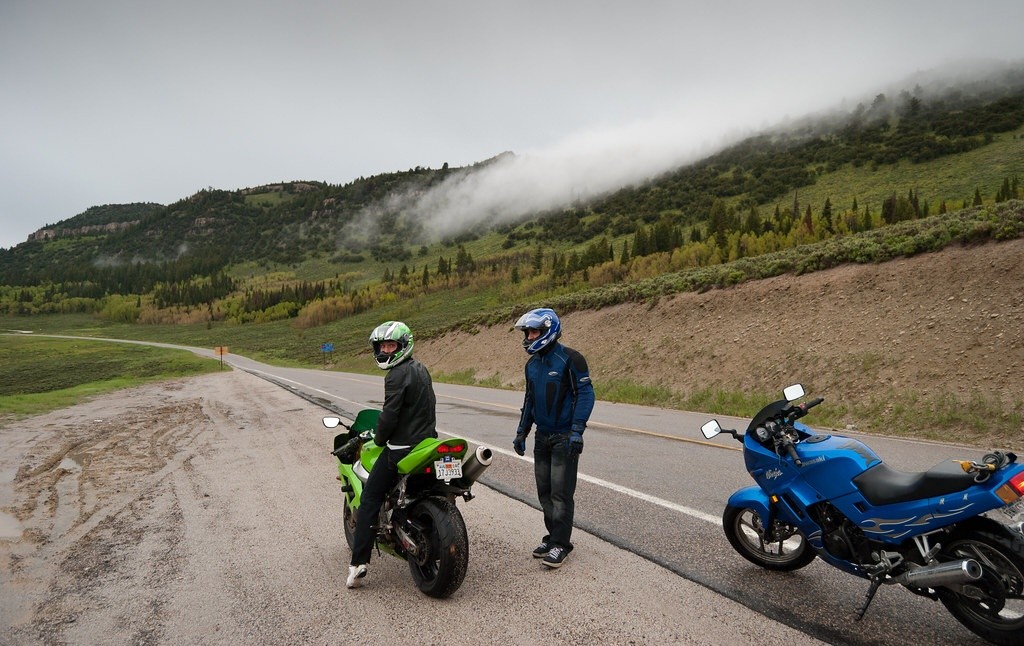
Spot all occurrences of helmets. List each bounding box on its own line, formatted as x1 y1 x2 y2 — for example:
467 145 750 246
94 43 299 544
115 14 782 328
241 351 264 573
520 308 562 355
368 320 415 370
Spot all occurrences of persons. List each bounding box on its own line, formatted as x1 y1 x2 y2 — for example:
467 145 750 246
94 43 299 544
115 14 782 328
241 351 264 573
344 320 437 589
513 309 596 568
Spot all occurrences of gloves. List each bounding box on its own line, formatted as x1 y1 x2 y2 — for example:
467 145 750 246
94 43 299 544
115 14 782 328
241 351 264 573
565 424 585 457
512 427 529 456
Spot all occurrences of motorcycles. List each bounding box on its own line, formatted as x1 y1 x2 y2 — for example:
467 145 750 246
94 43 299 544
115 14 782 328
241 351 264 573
322 408 495 598
700 383 1024 646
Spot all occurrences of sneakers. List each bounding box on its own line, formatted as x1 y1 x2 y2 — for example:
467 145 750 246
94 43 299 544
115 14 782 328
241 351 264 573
542 544 569 568
533 541 551 559
345 564 368 589
